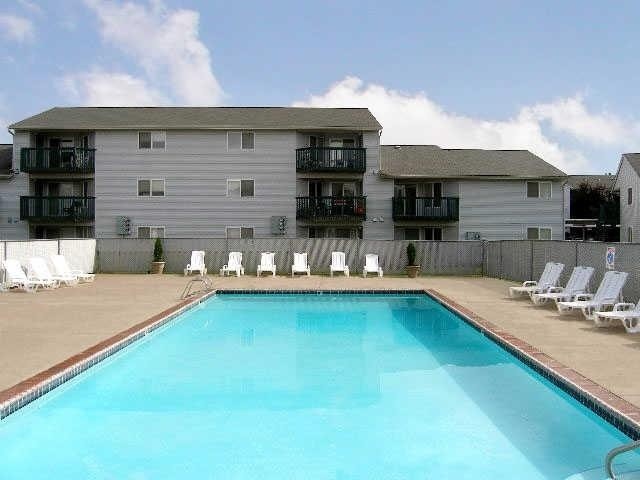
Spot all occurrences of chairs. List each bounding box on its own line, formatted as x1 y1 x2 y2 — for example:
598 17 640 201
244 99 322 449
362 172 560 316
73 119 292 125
330 251 349 278
363 253 383 278
0 254 95 291
257 252 276 277
220 251 244 276
292 252 310 277
183 251 207 276
509 262 640 335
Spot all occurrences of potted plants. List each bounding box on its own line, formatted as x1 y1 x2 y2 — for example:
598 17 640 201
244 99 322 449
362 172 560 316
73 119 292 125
405 243 421 278
150 237 166 274
